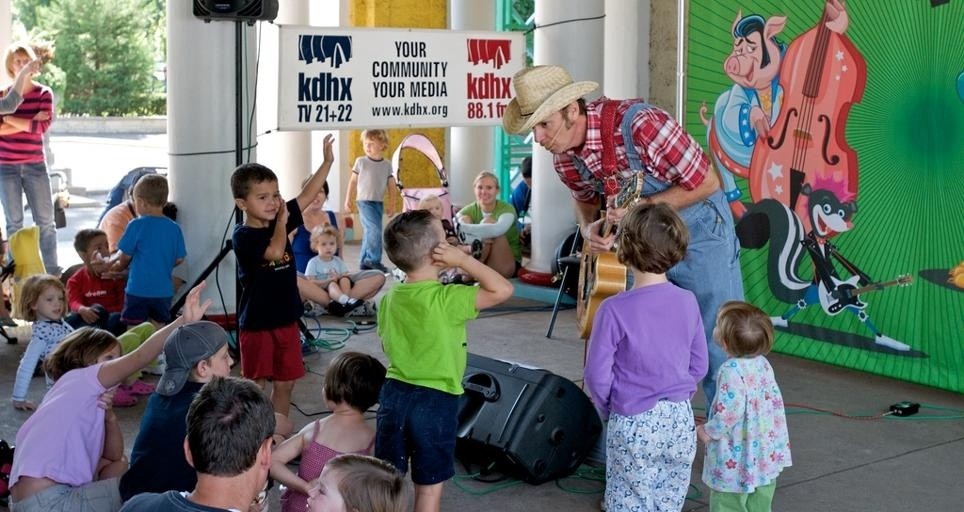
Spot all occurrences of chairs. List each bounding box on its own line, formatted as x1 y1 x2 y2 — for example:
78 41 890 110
545 220 587 339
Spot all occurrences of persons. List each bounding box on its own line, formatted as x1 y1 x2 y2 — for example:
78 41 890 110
269 353 388 511
288 174 385 319
375 210 515 512
695 301 793 512
61 227 127 336
8 279 215 512
102 173 185 334
1 58 45 113
0 44 67 276
305 224 366 311
452 172 521 279
96 169 141 254
14 275 158 412
585 203 709 512
118 320 234 504
229 133 336 466
500 65 744 467
305 453 408 512
511 155 532 251
115 375 278 511
346 129 397 272
418 194 483 285
1 225 48 344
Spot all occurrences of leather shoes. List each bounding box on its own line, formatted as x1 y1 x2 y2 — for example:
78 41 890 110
359 263 384 270
340 296 365 314
364 259 388 273
325 300 344 317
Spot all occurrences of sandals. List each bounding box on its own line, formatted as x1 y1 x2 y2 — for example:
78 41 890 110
112 387 140 408
119 380 157 397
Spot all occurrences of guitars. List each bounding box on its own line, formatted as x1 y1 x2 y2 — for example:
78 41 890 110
572 167 642 343
818 273 913 317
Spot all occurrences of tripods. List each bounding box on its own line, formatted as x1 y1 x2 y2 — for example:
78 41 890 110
171 26 316 341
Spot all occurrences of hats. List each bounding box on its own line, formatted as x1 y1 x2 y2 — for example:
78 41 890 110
155 320 231 398
500 63 600 137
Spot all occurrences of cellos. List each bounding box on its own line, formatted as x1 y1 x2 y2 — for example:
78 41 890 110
746 1 870 249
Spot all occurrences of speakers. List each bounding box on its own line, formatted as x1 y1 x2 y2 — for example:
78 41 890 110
454 351 603 486
192 0 279 26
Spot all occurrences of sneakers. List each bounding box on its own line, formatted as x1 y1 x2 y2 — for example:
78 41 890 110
441 274 479 286
367 299 376 316
303 299 327 318
